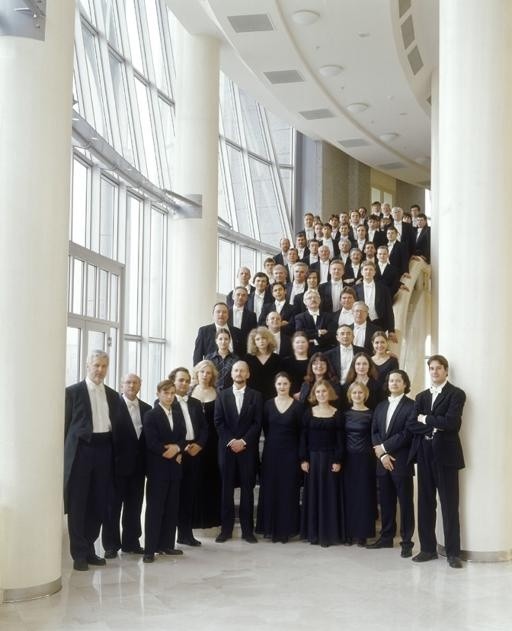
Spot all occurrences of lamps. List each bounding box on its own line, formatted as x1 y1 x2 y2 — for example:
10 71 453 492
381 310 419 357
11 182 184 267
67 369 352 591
320 65 342 77
348 104 368 114
380 133 398 143
293 11 319 26
415 156 429 166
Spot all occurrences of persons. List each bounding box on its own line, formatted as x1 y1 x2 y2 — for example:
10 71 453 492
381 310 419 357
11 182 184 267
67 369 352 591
254 371 305 544
188 360 222 529
154 367 208 546
367 369 415 558
226 201 431 403
297 379 346 547
141 380 187 562
200 330 242 388
64 350 125 571
193 302 244 365
213 360 264 543
406 355 466 568
102 374 152 559
342 381 375 546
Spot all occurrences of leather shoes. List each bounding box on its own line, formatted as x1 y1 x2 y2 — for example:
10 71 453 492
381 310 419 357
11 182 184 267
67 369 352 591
321 542 329 548
447 555 463 568
400 544 413 558
358 540 366 546
73 556 89 571
215 531 232 542
242 532 257 543
160 546 183 555
88 553 106 566
105 547 118 559
366 536 393 549
122 545 146 554
143 551 155 563
311 538 318 545
177 536 202 546
412 551 439 562
344 537 353 546
